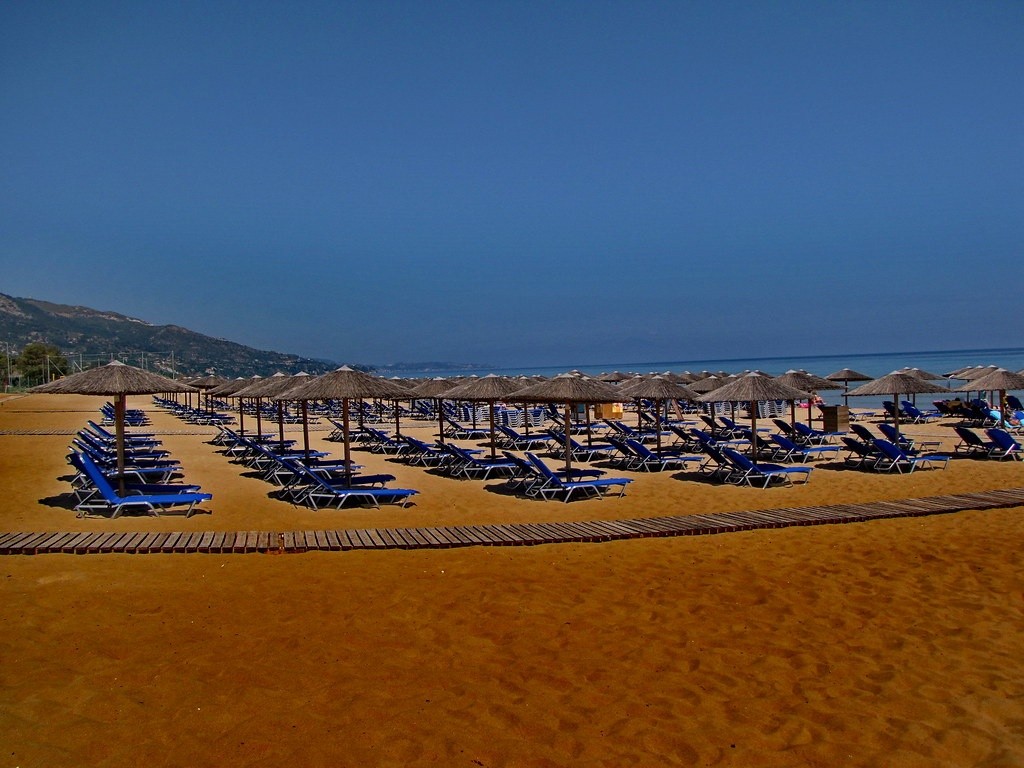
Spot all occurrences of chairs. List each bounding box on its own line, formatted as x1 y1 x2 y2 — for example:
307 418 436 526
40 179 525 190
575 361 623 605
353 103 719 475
66 394 1024 521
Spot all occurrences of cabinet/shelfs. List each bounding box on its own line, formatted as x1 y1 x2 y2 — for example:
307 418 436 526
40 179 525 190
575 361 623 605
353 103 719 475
594 403 624 421
823 406 850 435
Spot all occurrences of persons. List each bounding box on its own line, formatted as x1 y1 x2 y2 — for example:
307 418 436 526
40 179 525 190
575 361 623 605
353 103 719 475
812 391 824 404
983 399 1000 410
1004 401 1022 436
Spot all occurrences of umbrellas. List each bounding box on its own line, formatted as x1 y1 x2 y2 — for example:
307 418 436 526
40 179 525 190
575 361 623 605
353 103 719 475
26 361 1024 483
